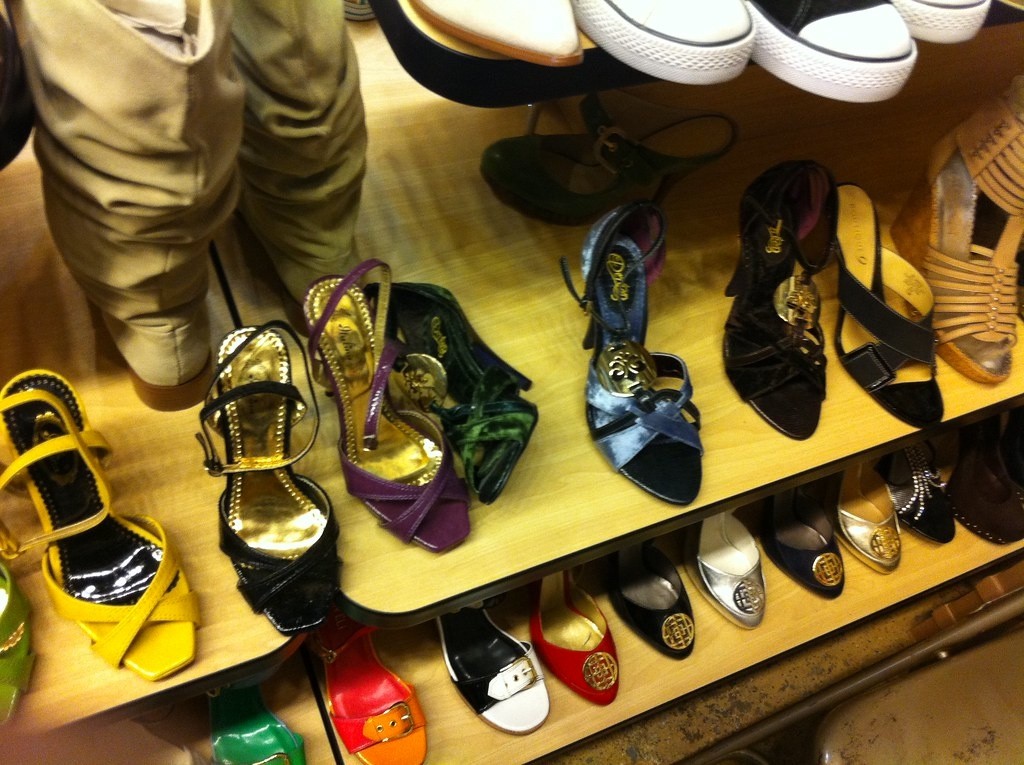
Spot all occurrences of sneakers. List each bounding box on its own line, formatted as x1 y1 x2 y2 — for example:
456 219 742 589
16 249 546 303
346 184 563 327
573 0 918 103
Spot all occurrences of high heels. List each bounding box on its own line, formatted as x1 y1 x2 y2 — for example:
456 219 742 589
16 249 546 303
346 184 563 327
562 200 703 505
362 280 538 506
481 93 738 225
299 259 472 553
195 320 343 632
722 159 837 441
825 182 943 428
892 74 1024 385
1 369 198 682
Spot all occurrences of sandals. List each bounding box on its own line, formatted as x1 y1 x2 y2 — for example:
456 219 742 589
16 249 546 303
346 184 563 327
0 565 36 727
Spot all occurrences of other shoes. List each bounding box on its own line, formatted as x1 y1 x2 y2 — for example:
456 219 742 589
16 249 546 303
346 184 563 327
202 407 1024 765
890 1 995 45
410 0 576 66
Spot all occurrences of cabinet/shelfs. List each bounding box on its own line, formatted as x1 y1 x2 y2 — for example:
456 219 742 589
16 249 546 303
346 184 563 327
0 19 1024 765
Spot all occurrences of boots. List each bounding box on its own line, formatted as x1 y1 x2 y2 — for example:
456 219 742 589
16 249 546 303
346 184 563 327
230 1 368 306
10 1 245 411
